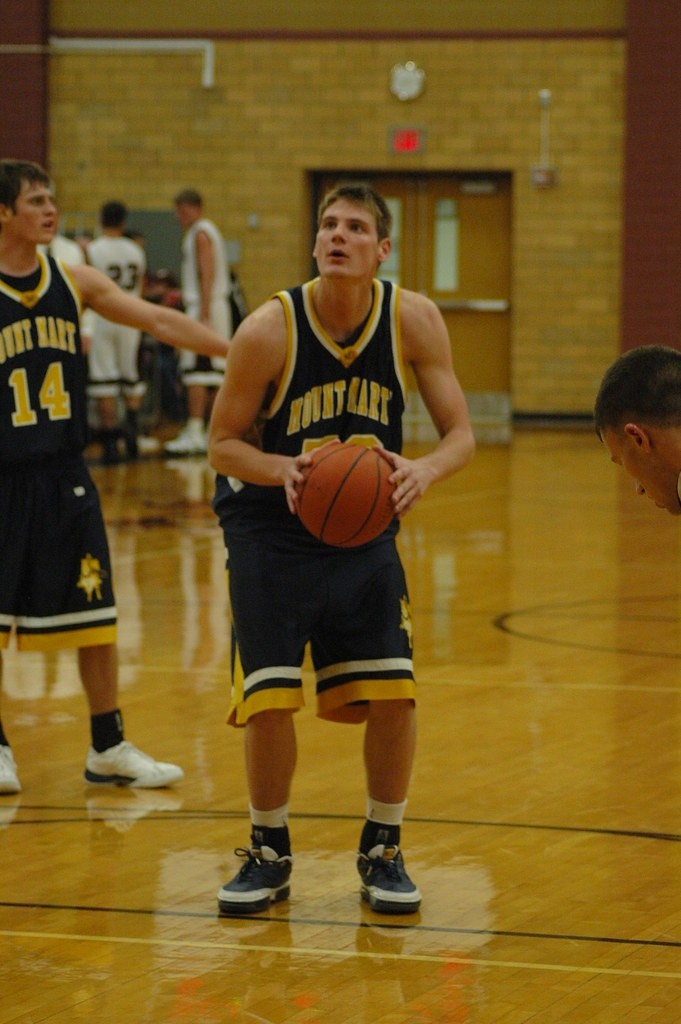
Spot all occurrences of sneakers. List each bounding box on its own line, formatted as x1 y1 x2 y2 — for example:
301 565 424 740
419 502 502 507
0 744 22 794
356 843 422 914
84 740 185 789
218 842 295 913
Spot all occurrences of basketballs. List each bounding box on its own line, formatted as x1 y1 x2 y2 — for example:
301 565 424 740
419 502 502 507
290 442 400 549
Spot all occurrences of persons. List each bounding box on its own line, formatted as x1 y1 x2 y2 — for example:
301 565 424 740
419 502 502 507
164 190 231 454
84 200 146 461
593 346 681 515
36 233 95 351
208 182 476 912
0 159 232 794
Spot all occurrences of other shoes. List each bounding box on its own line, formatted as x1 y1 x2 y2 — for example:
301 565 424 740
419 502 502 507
163 428 206 454
